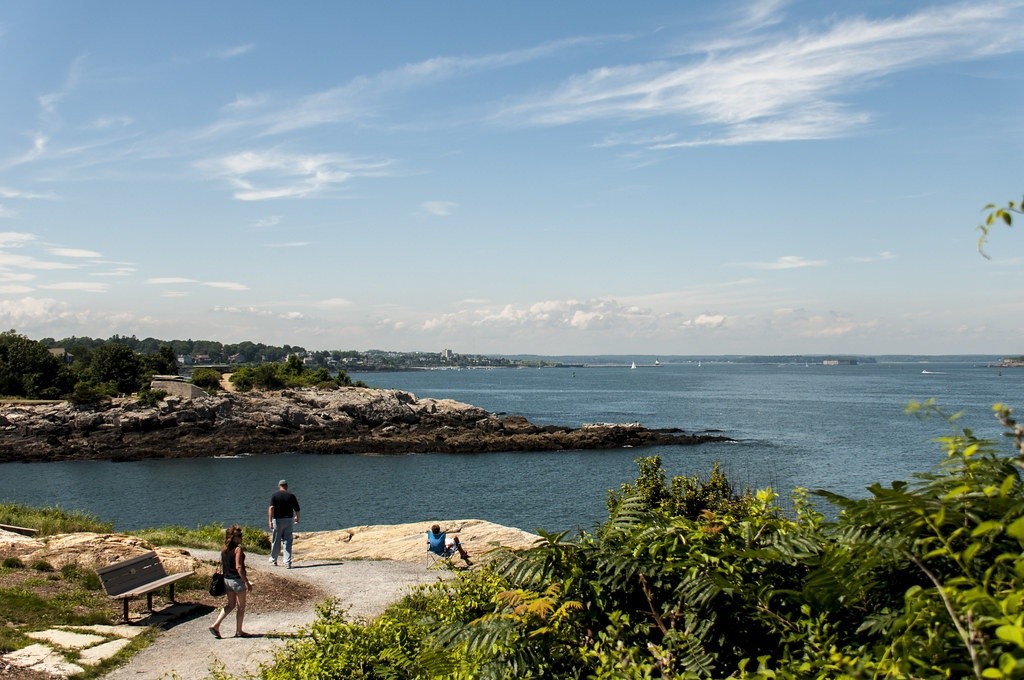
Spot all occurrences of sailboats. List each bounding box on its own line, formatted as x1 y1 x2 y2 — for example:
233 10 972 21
629 361 637 370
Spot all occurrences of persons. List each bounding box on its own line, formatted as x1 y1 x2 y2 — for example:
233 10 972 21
427 524 473 560
208 524 252 638
268 479 301 570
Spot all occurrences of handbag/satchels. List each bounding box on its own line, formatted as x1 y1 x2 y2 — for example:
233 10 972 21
209 572 226 597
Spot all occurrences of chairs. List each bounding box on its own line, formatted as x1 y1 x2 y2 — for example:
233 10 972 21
425 530 465 569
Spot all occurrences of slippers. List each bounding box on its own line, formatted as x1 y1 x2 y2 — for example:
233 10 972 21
234 632 248 637
209 627 222 638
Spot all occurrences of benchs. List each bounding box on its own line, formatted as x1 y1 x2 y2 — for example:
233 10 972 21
95 551 195 626
0 524 39 537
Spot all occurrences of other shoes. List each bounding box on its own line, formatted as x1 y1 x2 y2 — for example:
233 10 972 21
269 557 277 566
461 551 470 559
285 563 291 569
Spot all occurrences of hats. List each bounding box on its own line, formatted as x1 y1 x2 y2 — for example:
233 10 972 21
279 480 287 485
